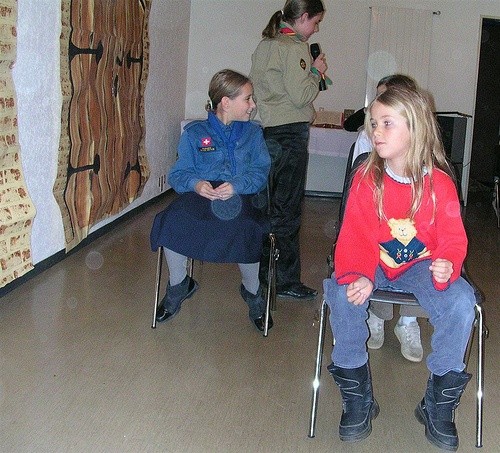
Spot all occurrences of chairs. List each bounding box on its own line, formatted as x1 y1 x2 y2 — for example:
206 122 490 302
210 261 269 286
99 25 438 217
151 139 293 337
308 151 490 448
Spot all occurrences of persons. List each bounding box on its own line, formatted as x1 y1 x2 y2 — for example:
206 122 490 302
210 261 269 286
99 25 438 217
324 87 476 453
249 0 327 299
352 73 424 362
155 70 276 332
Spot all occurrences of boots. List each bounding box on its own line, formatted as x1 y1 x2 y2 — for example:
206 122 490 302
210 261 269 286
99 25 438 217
327 360 380 442
414 369 472 451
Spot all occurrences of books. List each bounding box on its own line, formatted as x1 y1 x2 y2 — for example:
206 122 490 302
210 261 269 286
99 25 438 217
310 110 345 130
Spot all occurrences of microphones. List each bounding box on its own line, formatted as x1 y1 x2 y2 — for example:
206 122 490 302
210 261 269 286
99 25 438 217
309 43 326 91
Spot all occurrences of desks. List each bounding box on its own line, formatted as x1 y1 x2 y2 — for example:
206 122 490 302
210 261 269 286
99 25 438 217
305 127 359 198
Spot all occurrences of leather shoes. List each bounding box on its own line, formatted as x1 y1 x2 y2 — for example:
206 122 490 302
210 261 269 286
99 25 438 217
240 283 274 332
276 282 318 301
156 275 198 322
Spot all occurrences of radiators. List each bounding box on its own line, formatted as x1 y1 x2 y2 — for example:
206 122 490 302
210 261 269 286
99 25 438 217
364 5 441 108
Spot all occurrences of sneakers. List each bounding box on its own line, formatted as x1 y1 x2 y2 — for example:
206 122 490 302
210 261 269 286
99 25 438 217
366 308 384 349
394 315 424 362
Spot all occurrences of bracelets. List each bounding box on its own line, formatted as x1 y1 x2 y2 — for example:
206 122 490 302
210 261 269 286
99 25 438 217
310 67 332 85
